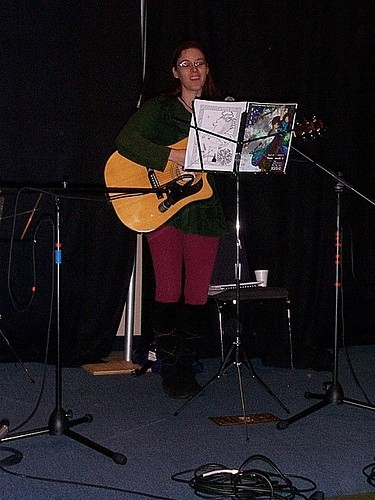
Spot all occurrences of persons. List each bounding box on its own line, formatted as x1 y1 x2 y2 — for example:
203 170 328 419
114 39 225 398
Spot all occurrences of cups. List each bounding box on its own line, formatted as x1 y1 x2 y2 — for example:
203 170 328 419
254 270 268 287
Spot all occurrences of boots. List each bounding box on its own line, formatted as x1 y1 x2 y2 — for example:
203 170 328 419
185 301 208 396
155 301 189 399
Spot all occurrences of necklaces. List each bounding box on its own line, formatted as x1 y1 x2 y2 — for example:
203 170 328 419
179 97 192 111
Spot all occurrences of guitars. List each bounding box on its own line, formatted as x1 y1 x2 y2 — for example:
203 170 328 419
104 114 325 232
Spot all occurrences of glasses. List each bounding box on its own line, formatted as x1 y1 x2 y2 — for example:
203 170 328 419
176 60 207 68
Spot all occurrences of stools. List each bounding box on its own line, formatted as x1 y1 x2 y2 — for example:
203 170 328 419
218 288 294 372
